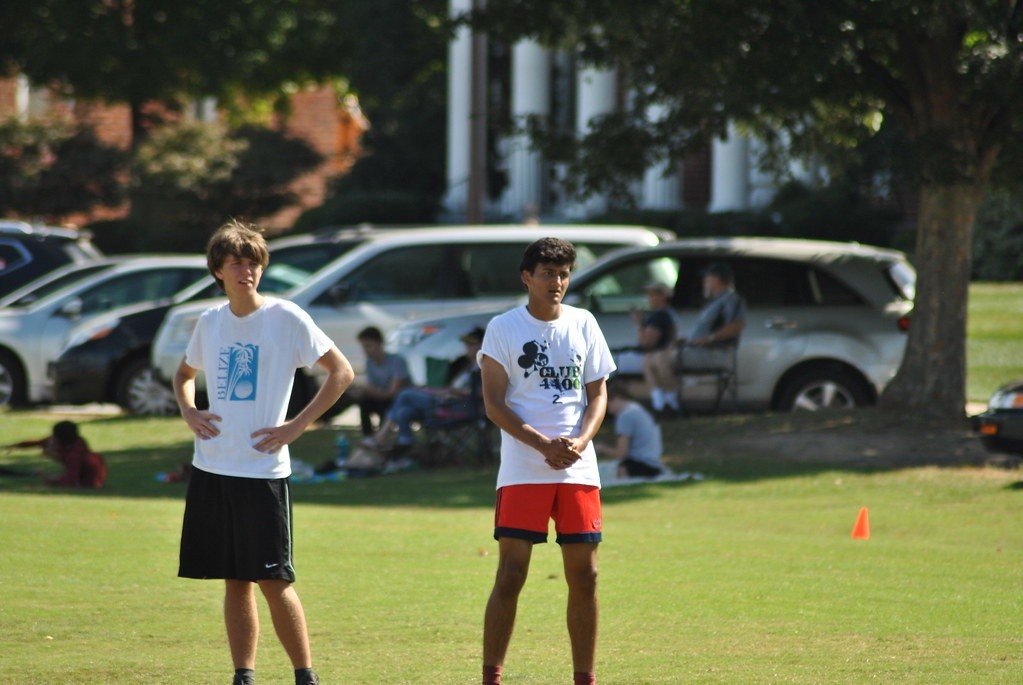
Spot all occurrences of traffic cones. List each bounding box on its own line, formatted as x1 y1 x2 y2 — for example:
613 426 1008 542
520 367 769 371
851 504 870 539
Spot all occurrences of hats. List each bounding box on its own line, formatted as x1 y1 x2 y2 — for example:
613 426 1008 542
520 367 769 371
646 281 673 299
459 328 486 342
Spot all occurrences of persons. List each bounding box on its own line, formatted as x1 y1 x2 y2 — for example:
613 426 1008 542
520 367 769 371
173 219 355 685
313 264 747 478
475 238 617 685
5 420 107 490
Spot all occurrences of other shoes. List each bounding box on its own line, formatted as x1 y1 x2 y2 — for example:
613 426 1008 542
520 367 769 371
362 438 393 451
651 402 690 421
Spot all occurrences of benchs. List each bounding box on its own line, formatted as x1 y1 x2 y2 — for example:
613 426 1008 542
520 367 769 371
608 332 739 416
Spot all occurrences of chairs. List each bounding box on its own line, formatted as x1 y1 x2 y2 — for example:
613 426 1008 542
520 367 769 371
369 357 495 469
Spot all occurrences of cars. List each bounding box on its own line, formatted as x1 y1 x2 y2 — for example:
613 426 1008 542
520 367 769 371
971 382 1023 458
380 235 916 418
0 220 398 424
152 224 687 426
